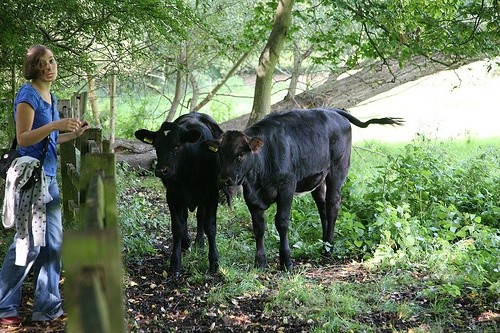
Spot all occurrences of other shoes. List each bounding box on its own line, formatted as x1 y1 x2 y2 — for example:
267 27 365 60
54 313 68 321
0 317 22 325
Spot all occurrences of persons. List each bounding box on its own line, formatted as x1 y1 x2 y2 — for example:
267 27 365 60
0 43 93 326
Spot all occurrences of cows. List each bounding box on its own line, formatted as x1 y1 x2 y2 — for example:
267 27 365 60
199 107 408 275
135 113 232 277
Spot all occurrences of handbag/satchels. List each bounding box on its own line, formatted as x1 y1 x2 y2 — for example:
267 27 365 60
0 150 41 190
